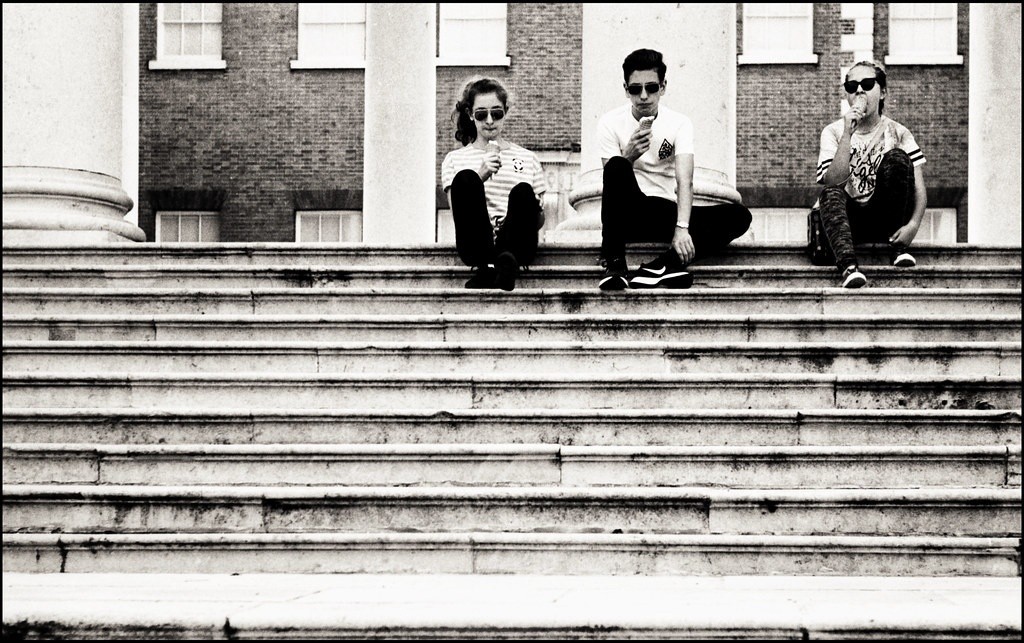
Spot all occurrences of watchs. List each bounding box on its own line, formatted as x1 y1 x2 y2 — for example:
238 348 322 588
675 220 689 229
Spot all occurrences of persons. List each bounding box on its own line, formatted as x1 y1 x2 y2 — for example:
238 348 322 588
598 48 752 290
816 60 927 288
441 78 547 291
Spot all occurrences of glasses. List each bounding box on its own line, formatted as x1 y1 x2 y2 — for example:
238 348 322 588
843 77 878 93
626 81 660 95
475 109 504 121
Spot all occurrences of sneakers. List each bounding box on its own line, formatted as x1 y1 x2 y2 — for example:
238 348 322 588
890 246 916 266
598 257 629 289
629 265 692 288
841 265 866 288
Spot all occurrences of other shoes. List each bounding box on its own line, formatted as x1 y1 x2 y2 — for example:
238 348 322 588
465 263 496 288
496 252 516 292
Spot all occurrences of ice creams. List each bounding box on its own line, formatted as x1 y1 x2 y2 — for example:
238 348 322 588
639 115 655 131
850 98 867 129
485 140 501 180
